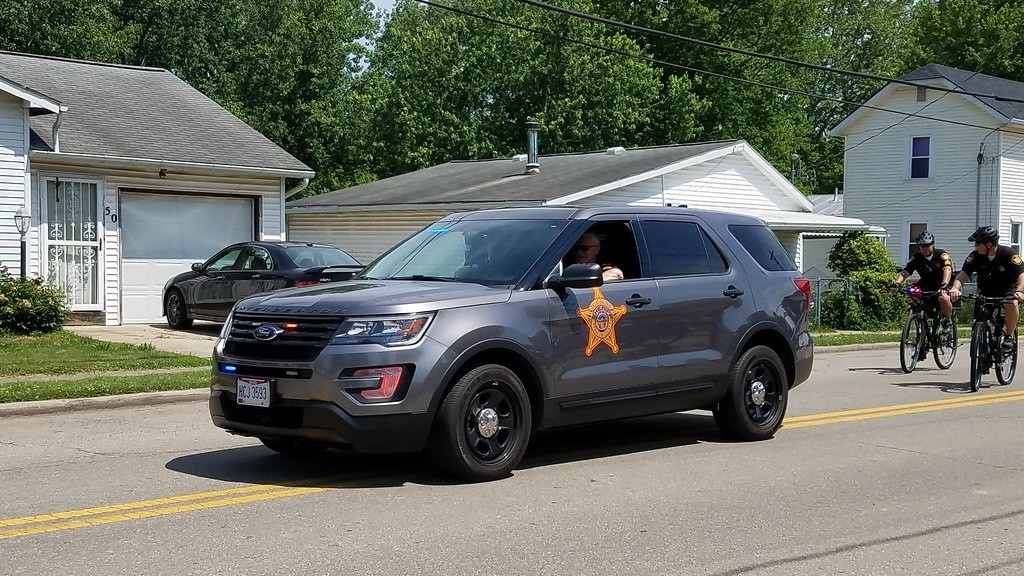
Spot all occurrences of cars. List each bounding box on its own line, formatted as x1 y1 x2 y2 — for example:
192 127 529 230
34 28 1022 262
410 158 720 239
159 239 372 330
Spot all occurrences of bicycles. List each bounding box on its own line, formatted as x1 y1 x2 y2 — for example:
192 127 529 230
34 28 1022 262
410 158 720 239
893 284 962 373
946 290 1024 392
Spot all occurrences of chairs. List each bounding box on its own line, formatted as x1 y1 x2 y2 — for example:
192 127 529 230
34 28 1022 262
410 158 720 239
300 258 313 268
251 254 268 270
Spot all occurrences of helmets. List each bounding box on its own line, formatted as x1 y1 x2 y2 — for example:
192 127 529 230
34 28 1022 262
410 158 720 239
915 231 935 245
967 225 1000 245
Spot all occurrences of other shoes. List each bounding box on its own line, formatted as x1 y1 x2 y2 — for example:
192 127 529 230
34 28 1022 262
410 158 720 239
1001 334 1016 348
911 353 926 360
939 318 954 341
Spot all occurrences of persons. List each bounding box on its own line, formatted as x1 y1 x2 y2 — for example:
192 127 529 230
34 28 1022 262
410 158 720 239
454 238 502 280
948 226 1024 375
574 233 624 283
890 230 953 359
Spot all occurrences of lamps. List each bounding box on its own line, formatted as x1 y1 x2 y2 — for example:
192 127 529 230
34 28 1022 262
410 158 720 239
14 202 32 236
158 168 167 179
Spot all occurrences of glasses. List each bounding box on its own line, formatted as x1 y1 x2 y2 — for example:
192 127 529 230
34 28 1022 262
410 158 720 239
573 245 595 252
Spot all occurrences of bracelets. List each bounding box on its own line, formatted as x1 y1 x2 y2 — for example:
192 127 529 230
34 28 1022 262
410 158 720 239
1014 291 1024 295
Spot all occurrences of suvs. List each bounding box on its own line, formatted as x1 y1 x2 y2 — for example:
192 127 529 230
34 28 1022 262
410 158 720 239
208 200 816 483
